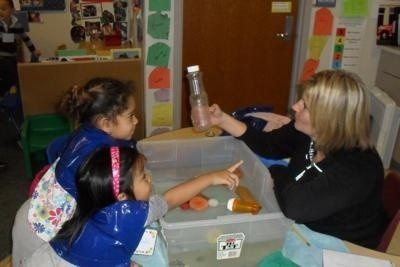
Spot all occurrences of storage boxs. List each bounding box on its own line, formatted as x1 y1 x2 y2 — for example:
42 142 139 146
135 135 287 254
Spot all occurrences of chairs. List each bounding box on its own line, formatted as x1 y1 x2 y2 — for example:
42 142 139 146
344 169 400 265
21 111 74 178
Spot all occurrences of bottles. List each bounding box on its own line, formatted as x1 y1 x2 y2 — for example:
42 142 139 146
186 64 213 133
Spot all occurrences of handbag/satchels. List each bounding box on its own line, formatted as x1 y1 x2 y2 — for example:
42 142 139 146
26 157 78 242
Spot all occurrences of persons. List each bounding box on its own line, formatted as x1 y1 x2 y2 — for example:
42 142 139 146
22 146 244 267
191 69 384 250
11 77 139 267
0 0 42 150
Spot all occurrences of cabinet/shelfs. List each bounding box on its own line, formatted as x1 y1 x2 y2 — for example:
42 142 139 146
372 46 400 165
17 57 144 140
